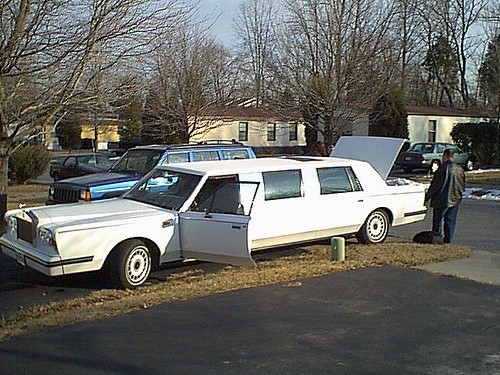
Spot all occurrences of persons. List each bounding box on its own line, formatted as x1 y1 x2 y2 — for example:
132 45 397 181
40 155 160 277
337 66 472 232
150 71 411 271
424 149 466 243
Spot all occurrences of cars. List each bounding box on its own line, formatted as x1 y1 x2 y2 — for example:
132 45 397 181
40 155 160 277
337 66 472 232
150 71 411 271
1 136 428 288
49 154 114 183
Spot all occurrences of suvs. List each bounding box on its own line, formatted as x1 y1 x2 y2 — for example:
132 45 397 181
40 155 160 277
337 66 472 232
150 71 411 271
400 141 478 172
49 139 255 205
